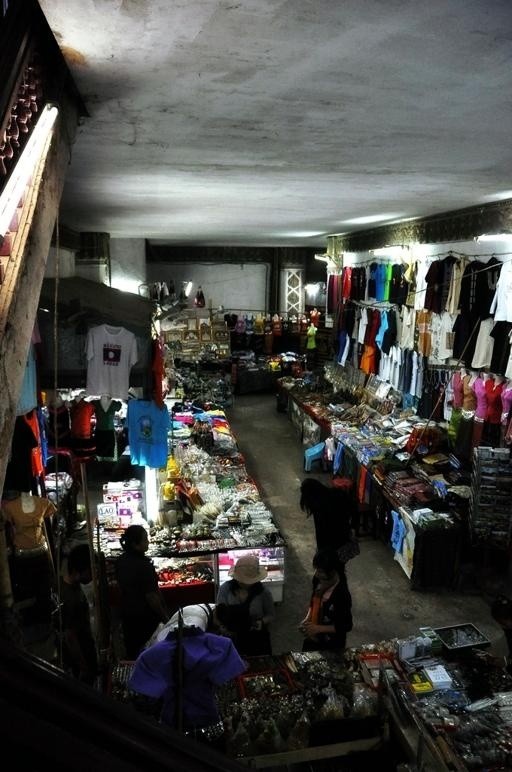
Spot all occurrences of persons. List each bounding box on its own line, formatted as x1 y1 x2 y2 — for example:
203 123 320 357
217 562 279 655
306 323 318 349
224 311 310 335
117 525 170 659
300 477 360 569
146 599 250 638
451 368 512 427
488 596 511 668
63 543 111 673
310 308 321 327
298 550 353 651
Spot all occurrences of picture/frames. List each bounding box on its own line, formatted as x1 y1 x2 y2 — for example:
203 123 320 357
165 317 230 359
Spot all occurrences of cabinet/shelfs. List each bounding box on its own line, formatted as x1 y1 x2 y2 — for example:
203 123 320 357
0 370 512 772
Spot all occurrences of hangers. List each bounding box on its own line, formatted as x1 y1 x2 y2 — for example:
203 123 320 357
354 250 497 269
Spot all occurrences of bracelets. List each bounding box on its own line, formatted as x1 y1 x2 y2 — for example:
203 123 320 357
503 655 507 666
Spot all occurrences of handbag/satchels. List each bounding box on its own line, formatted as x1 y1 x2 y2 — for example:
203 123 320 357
338 542 360 564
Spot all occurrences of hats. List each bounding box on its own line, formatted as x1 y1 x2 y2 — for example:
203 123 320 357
227 556 268 584
